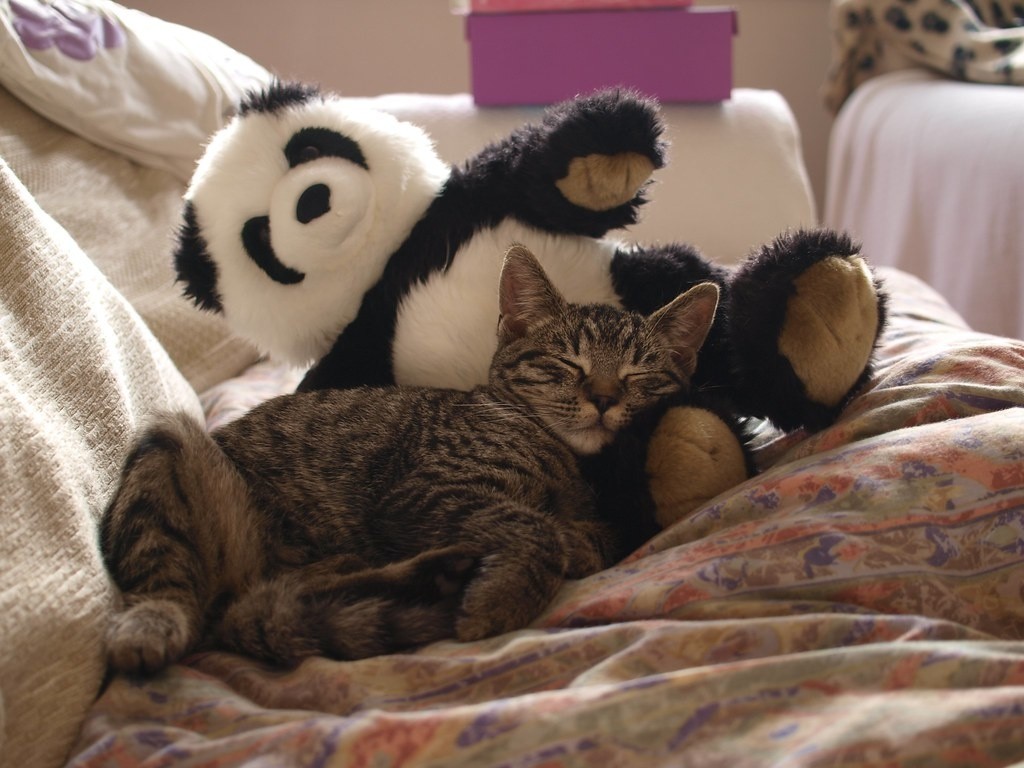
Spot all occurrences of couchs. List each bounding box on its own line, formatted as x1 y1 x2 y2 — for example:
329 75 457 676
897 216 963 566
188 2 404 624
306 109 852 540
0 0 1024 768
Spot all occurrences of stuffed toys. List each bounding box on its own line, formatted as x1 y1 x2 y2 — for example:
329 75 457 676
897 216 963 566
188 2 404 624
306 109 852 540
172 79 886 538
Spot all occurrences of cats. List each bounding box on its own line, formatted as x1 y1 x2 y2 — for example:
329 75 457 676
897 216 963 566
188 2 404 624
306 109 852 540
96 240 720 676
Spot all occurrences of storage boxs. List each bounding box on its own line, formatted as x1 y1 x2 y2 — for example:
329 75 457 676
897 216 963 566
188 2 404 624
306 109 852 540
459 7 740 107
